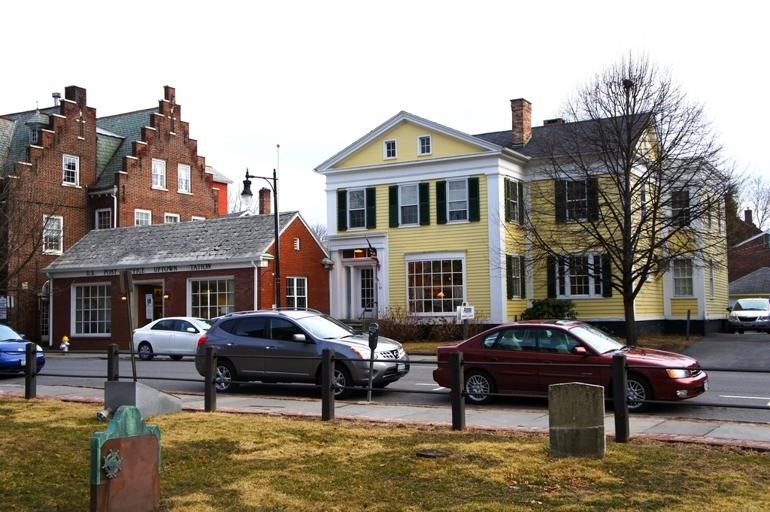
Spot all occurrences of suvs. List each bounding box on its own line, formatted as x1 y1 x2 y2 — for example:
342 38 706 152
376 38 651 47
193 306 411 400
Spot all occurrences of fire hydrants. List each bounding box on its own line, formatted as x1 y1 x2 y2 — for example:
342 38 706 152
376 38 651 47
59 335 70 353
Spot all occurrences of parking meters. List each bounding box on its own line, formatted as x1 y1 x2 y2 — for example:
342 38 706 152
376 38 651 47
366 322 379 401
456 301 474 340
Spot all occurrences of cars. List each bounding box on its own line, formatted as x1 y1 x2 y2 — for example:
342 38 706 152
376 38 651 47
129 316 214 362
0 324 46 377
432 318 710 413
724 297 770 335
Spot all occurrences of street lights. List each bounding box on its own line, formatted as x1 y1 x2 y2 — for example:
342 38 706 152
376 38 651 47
240 167 281 312
